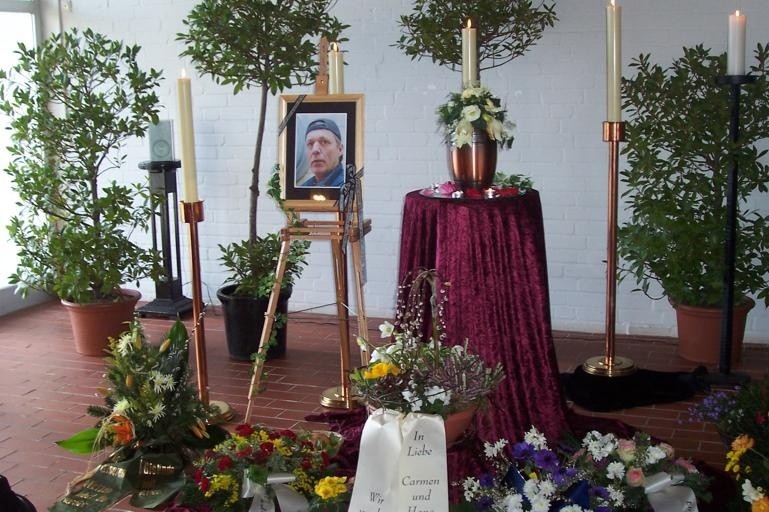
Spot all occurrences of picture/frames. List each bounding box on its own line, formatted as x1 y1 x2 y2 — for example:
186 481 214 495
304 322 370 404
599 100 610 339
278 95 363 210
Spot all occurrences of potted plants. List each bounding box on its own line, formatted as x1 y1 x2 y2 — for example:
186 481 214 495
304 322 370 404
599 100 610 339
175 1 351 360
0 27 169 355
603 42 769 364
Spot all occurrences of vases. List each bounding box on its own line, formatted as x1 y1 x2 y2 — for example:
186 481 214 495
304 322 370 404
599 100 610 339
451 128 497 191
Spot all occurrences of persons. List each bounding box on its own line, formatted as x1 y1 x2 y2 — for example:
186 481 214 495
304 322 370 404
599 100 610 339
298 117 344 187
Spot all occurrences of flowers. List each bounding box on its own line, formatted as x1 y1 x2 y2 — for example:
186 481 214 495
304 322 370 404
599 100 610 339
434 81 516 150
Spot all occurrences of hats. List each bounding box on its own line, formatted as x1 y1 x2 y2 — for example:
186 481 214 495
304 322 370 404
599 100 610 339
305 119 341 141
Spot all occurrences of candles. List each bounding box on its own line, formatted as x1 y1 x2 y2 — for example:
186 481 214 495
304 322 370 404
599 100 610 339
328 42 344 95
606 0 622 122
727 11 746 75
177 68 199 203
462 19 477 82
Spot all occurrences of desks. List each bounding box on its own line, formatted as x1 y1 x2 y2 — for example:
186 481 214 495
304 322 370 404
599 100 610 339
391 185 572 476
